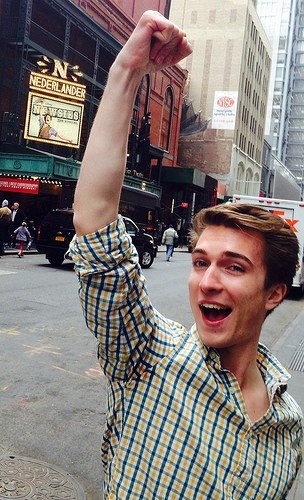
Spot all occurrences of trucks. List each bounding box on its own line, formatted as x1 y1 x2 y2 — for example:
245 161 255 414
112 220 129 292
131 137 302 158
231 194 304 301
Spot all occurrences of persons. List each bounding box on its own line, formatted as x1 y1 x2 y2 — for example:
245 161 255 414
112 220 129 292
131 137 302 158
0 199 186 262
62 9 304 500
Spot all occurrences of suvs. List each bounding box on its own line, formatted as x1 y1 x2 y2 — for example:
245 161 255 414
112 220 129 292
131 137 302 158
34 207 158 269
